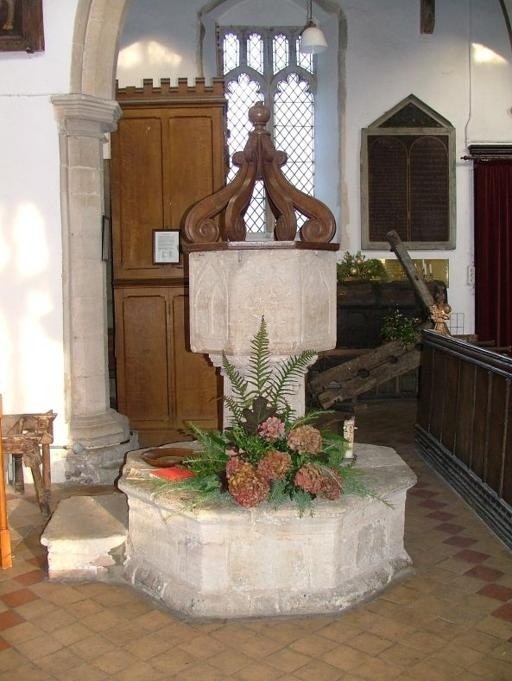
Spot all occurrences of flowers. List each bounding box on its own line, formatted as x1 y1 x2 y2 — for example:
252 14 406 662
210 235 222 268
152 312 397 520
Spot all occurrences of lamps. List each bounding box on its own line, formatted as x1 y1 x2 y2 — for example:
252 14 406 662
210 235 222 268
299 0 328 54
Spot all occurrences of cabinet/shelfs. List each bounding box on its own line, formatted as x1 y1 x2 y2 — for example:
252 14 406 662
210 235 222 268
112 285 226 432
109 75 226 286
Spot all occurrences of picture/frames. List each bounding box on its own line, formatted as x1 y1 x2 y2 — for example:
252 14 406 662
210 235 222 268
0 0 46 53
